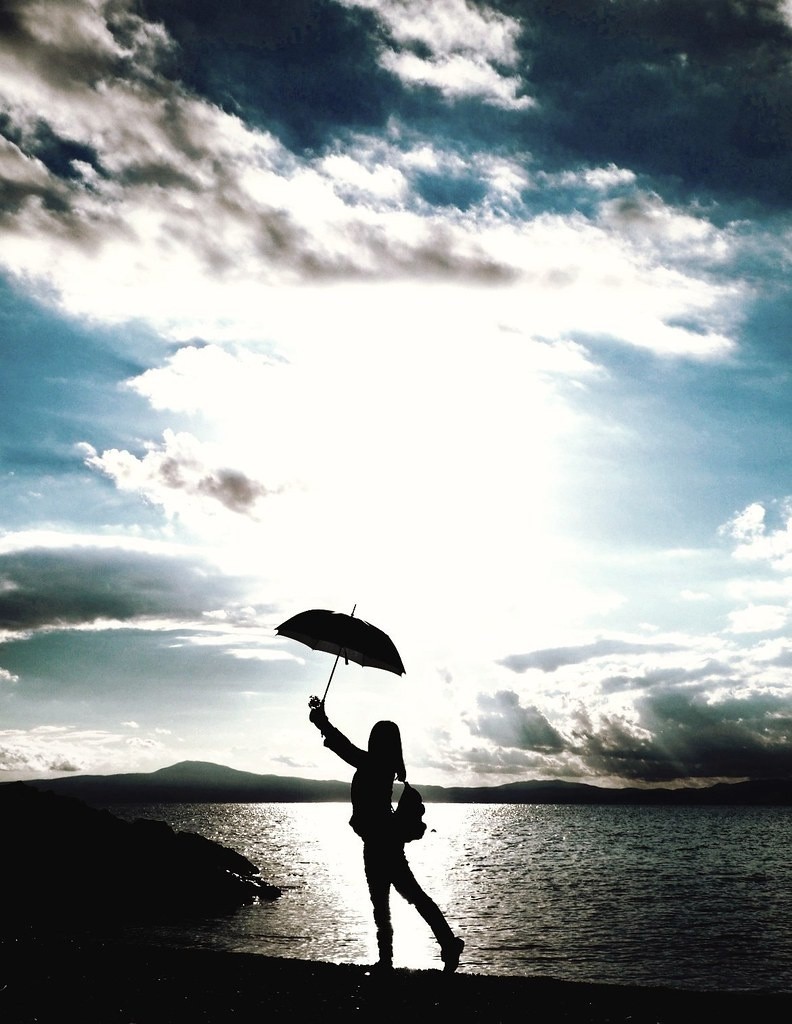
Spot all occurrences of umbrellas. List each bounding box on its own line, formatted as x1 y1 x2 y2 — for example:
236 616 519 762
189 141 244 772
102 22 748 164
274 603 406 706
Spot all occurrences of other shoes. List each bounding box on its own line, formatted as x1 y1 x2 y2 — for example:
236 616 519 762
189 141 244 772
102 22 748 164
442 937 465 975
366 961 392 976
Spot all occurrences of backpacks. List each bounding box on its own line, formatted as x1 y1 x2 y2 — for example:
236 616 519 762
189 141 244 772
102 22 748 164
385 782 427 842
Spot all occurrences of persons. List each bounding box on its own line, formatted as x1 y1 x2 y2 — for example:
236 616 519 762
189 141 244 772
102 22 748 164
309 701 464 974
